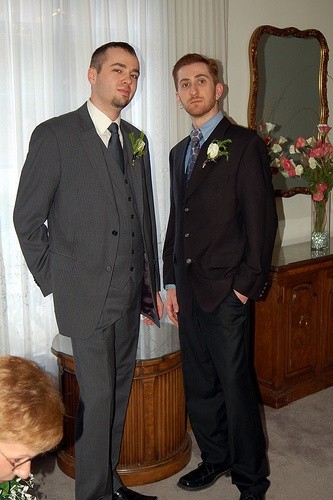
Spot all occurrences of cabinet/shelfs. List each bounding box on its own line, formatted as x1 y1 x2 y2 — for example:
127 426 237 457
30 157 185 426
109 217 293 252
256 236 333 410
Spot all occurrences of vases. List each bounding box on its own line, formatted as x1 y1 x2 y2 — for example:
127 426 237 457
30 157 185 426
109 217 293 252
311 232 329 250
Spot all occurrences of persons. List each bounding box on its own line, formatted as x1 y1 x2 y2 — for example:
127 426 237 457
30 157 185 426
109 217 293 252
162 53 278 500
0 354 64 484
13 41 165 500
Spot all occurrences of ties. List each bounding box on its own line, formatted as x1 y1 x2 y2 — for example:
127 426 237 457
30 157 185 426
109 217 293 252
186 129 202 184
107 123 125 174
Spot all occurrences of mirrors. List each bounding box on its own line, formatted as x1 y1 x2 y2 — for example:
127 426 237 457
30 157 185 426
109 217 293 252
246 25 329 199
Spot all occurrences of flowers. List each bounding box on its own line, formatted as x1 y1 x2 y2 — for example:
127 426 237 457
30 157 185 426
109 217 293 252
258 123 295 179
295 123 332 231
202 138 232 169
128 130 147 165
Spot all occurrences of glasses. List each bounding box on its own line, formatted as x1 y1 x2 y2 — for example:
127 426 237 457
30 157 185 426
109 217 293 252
0 452 33 471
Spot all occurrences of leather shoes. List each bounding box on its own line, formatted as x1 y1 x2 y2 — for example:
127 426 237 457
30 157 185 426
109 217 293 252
177 461 233 493
111 486 158 500
239 487 266 500
